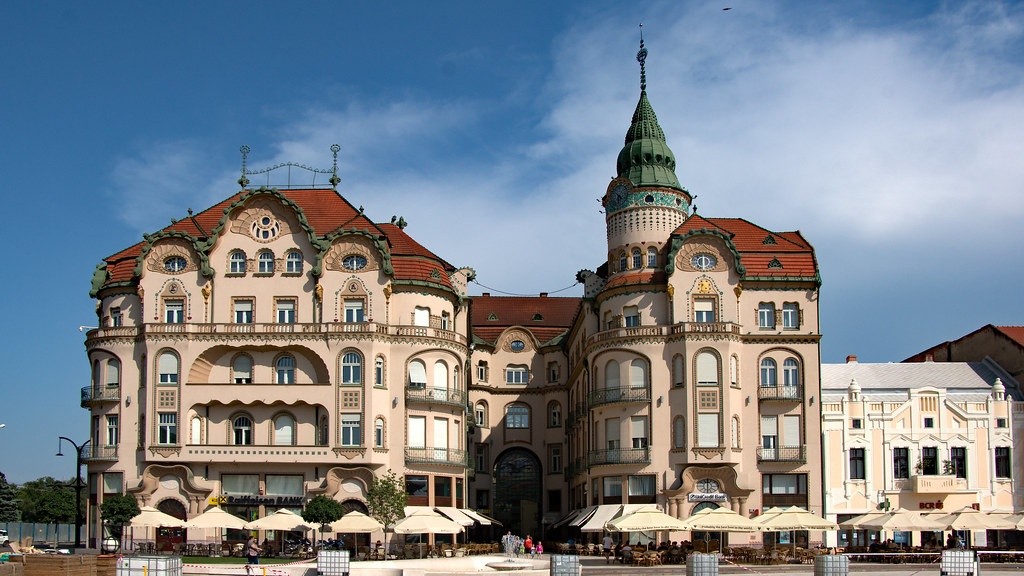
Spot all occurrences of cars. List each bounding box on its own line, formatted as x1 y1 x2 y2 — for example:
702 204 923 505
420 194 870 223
0 529 10 547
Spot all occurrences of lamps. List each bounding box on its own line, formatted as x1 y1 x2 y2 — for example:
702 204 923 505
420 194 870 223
127 395 131 403
395 397 398 405
812 396 815 403
748 395 751 403
679 320 684 323
660 396 664 403
748 331 752 334
810 331 814 333
779 331 782 333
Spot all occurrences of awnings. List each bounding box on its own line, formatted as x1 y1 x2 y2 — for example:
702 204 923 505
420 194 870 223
547 511 579 529
581 505 622 532
461 510 491 525
623 504 656 517
568 506 598 526
436 508 475 526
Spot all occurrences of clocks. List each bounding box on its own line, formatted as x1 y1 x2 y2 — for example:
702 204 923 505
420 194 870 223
609 185 628 207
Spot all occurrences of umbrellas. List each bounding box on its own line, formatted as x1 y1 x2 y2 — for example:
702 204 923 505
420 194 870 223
182 507 248 554
243 509 314 554
605 505 763 554
128 504 186 553
385 507 466 559
934 505 1024 550
750 505 947 557
313 510 384 557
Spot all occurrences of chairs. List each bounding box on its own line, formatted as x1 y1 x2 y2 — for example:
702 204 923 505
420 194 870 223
555 542 1024 567
138 542 499 562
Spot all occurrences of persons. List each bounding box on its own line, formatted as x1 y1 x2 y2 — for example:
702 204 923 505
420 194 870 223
602 533 613 565
869 538 898 562
614 540 645 564
246 536 262 565
514 535 543 559
647 539 694 564
947 534 956 549
935 540 945 548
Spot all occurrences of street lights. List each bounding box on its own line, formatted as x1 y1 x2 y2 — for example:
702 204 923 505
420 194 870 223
56 436 91 548
